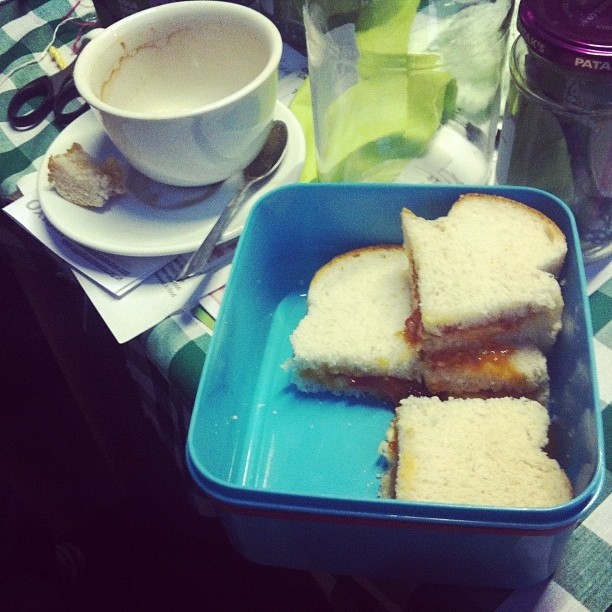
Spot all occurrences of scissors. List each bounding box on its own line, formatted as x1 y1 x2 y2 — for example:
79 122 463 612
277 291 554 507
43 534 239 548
8 39 90 128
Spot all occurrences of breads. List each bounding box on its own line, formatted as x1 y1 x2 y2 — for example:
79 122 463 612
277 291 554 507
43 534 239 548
373 394 576 509
398 193 568 348
46 141 127 212
289 245 551 408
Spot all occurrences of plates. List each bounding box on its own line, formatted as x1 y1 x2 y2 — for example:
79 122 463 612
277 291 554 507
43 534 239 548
37 99 307 260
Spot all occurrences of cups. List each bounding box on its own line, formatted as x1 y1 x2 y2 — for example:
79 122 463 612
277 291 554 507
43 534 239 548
72 1 283 190
494 34 612 266
302 0 515 188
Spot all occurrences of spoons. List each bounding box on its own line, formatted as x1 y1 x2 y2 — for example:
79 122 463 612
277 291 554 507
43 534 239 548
173 120 290 282
